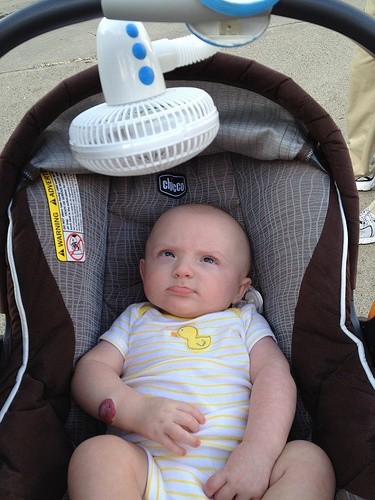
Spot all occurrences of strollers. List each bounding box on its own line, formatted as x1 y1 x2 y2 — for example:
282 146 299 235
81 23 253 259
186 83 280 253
0 0 375 500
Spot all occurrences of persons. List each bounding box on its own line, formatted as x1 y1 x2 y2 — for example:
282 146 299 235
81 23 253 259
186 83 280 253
67 204 336 500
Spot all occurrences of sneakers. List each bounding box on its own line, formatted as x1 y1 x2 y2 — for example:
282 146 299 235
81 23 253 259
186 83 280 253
356 173 375 191
358 209 375 244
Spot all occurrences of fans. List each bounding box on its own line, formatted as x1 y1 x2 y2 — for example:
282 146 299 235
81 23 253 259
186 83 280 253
67 0 281 177
